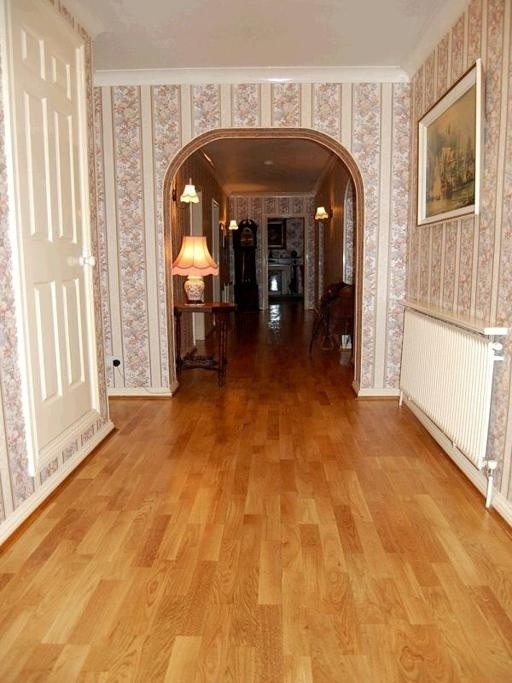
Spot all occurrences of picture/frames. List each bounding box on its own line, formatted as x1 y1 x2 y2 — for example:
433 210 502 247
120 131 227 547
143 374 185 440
415 55 483 226
268 218 286 249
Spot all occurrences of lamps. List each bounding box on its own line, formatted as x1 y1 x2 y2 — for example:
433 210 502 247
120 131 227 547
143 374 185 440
314 206 333 224
225 218 239 235
172 176 200 209
173 234 219 304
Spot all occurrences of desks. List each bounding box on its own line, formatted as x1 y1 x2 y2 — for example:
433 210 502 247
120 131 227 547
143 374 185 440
175 301 238 387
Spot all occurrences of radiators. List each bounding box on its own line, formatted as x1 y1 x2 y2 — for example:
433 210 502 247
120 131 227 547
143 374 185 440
397 307 502 508
